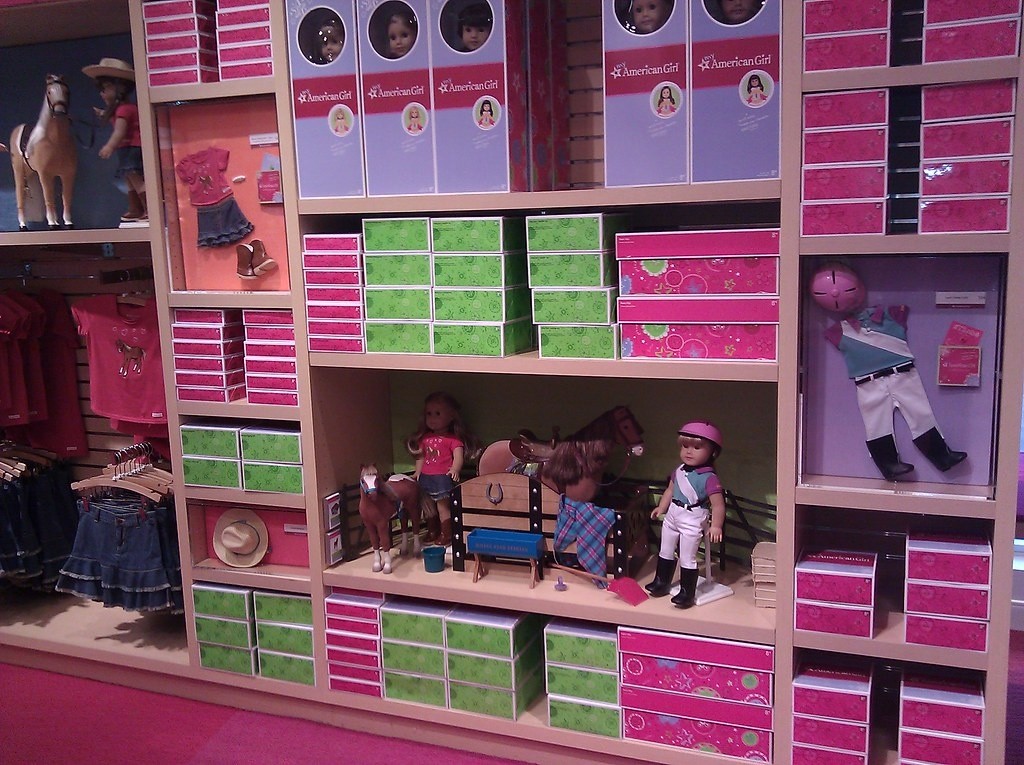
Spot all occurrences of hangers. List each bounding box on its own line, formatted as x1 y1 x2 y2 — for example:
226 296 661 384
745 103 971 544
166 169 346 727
71 442 174 512
116 268 151 306
0 437 58 482
12 272 42 295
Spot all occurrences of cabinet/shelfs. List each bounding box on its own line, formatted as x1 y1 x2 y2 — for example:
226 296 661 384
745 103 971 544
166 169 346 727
0 0 1024 765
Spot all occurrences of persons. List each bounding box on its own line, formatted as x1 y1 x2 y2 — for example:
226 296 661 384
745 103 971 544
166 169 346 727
645 422 725 608
404 391 479 547
387 13 417 58
631 0 668 32
317 19 344 61
81 59 149 221
717 0 759 23
458 3 493 50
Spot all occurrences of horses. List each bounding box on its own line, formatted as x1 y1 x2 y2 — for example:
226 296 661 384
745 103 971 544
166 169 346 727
478 406 645 503
0 72 78 231
358 461 422 574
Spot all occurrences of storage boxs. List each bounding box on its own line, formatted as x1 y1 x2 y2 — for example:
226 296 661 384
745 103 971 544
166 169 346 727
181 421 304 496
301 233 368 353
285 0 568 200
795 546 881 640
171 308 299 406
191 581 316 687
898 668 984 764
905 523 992 651
791 653 876 765
617 625 774 765
141 0 273 86
544 617 623 739
601 0 783 188
615 224 782 363
801 0 1022 237
324 585 553 721
362 212 634 361
